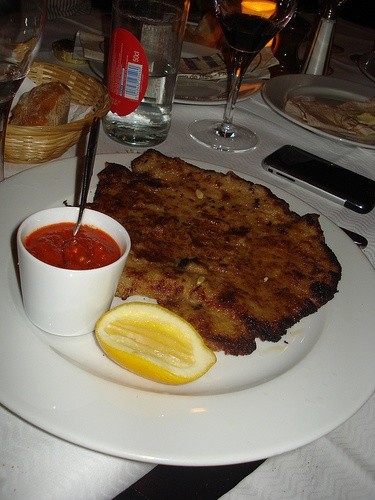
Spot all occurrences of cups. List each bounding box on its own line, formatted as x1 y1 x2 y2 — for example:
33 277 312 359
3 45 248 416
102 0 181 147
17 207 131 337
0 7 45 179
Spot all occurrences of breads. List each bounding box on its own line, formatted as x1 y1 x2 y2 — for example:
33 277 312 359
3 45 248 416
196 13 224 43
8 81 71 127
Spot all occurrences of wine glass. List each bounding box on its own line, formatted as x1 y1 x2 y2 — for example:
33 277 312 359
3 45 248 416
189 0 297 152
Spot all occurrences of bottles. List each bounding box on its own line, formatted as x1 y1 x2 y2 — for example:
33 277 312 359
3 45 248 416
297 5 336 76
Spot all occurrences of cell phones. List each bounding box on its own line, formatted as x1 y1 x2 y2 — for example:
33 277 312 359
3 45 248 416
261 144 375 214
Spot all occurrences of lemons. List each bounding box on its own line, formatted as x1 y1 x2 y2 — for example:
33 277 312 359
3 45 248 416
95 301 218 384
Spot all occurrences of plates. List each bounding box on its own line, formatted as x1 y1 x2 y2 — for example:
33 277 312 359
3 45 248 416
90 21 266 105
359 50 375 81
262 74 375 152
0 154 375 466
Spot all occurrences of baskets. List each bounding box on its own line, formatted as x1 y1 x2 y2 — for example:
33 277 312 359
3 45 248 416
3 61 110 164
